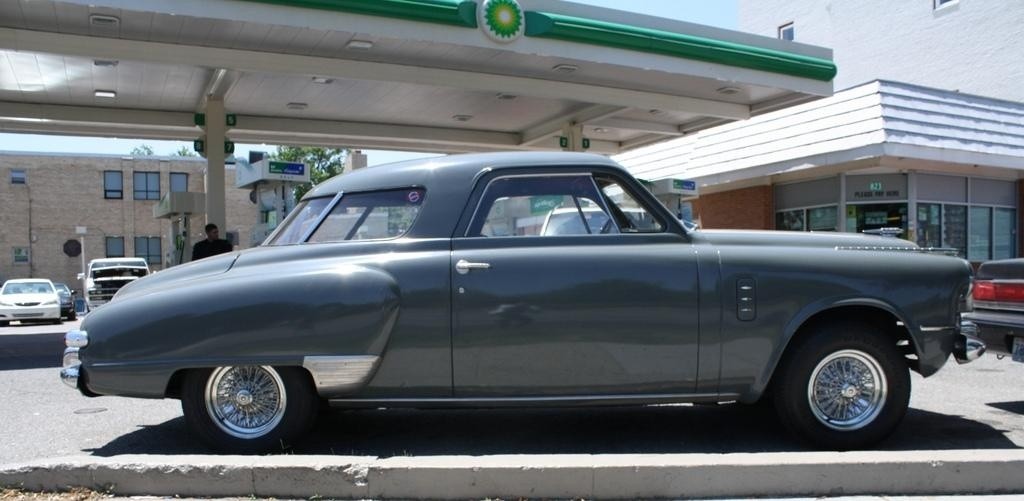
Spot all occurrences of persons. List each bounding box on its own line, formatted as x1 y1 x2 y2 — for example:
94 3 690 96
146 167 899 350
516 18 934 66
192 223 232 261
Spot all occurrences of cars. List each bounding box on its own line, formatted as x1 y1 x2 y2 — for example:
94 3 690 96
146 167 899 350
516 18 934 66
539 203 655 237
0 255 157 326
970 251 1024 363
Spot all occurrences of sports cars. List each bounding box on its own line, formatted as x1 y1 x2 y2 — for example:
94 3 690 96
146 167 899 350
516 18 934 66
55 148 993 449
